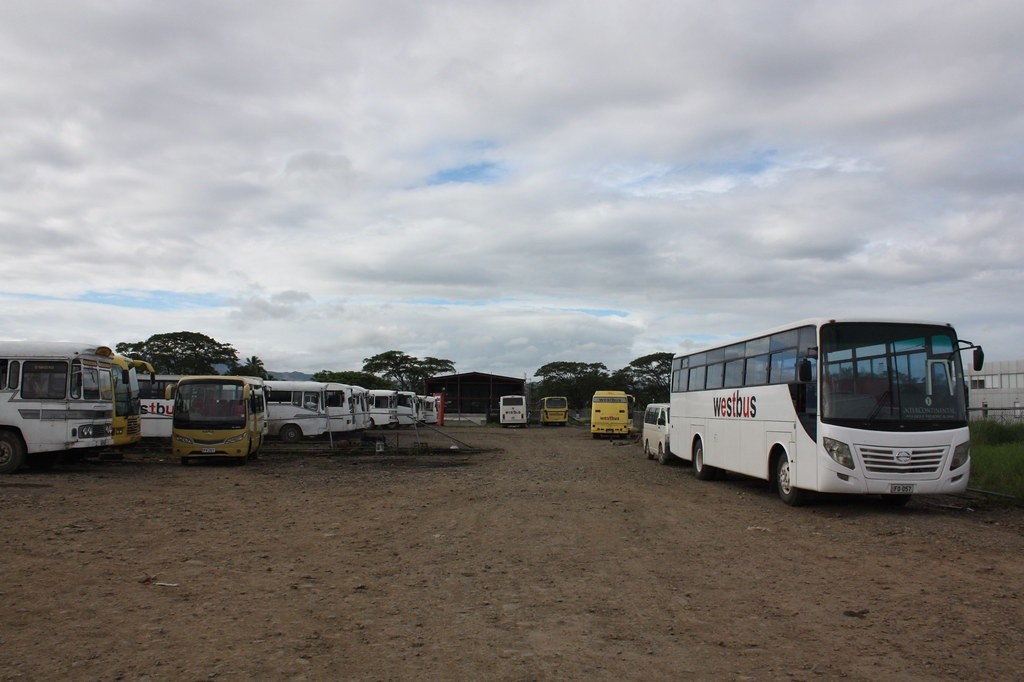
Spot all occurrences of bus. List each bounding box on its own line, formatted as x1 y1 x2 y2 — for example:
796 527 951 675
266 379 443 445
667 314 985 506
164 375 268 466
114 354 156 447
0 339 115 473
139 373 175 437
535 396 569 427
590 389 635 440
499 395 528 429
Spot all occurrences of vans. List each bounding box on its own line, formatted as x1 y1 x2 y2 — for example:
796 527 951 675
642 402 672 466
